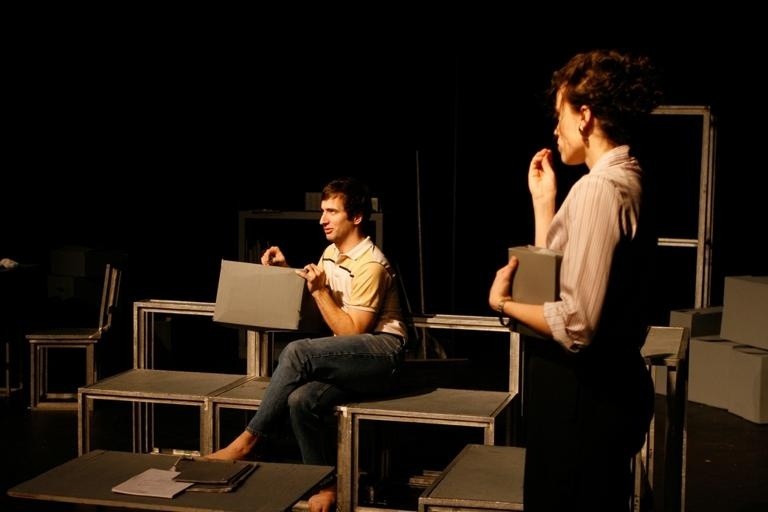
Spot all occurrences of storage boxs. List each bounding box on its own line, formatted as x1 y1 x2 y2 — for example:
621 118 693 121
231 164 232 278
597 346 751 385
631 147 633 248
688 337 725 409
718 274 768 351
725 344 767 423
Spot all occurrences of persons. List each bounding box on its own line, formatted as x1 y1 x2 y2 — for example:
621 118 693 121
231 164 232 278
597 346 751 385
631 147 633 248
206 179 411 511
486 50 669 511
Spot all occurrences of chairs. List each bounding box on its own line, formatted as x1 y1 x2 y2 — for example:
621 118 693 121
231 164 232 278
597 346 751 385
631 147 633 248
417 364 673 512
208 329 273 458
333 314 526 512
74 299 261 459
23 262 120 411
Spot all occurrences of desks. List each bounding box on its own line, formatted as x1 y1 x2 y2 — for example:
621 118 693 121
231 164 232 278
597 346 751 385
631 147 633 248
1 447 337 512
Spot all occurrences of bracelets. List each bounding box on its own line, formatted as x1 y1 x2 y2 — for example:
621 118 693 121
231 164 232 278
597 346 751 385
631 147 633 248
497 297 514 314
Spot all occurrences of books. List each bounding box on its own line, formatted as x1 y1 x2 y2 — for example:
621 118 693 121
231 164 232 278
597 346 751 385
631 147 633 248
110 452 259 500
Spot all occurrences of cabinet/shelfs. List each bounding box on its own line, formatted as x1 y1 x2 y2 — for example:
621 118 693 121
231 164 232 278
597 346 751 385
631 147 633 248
646 103 718 310
